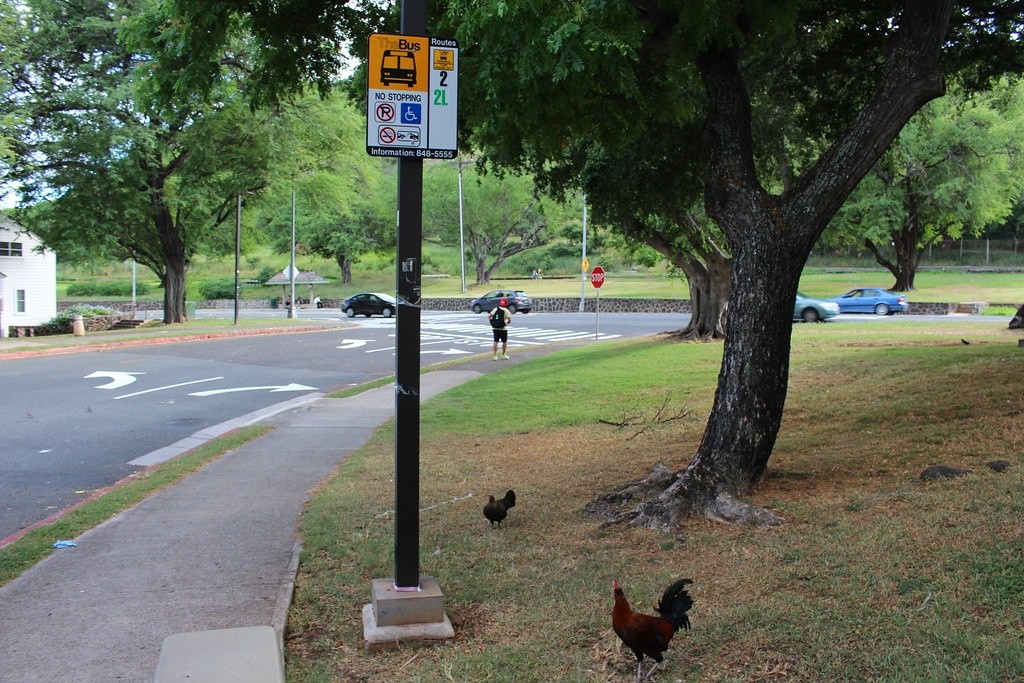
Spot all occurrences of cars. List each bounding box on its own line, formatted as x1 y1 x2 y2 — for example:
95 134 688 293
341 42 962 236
792 291 841 323
339 293 395 319
470 289 533 313
822 286 909 316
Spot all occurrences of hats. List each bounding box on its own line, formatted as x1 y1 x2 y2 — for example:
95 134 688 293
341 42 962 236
499 298 508 306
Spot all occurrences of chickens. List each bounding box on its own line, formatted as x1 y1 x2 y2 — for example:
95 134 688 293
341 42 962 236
483 489 516 530
612 578 694 683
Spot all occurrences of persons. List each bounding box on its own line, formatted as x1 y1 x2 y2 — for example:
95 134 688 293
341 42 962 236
488 298 510 361
271 296 282 308
314 295 323 308
537 267 544 281
286 294 290 305
296 297 304 305
532 270 538 282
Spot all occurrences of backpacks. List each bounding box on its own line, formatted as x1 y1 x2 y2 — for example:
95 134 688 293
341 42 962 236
490 308 506 328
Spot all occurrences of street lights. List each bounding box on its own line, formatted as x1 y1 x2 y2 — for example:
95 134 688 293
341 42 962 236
235 190 255 325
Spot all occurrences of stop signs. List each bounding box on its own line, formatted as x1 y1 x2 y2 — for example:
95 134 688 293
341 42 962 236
590 266 604 289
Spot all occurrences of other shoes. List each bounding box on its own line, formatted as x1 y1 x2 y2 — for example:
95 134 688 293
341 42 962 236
493 355 498 360
502 355 511 360
